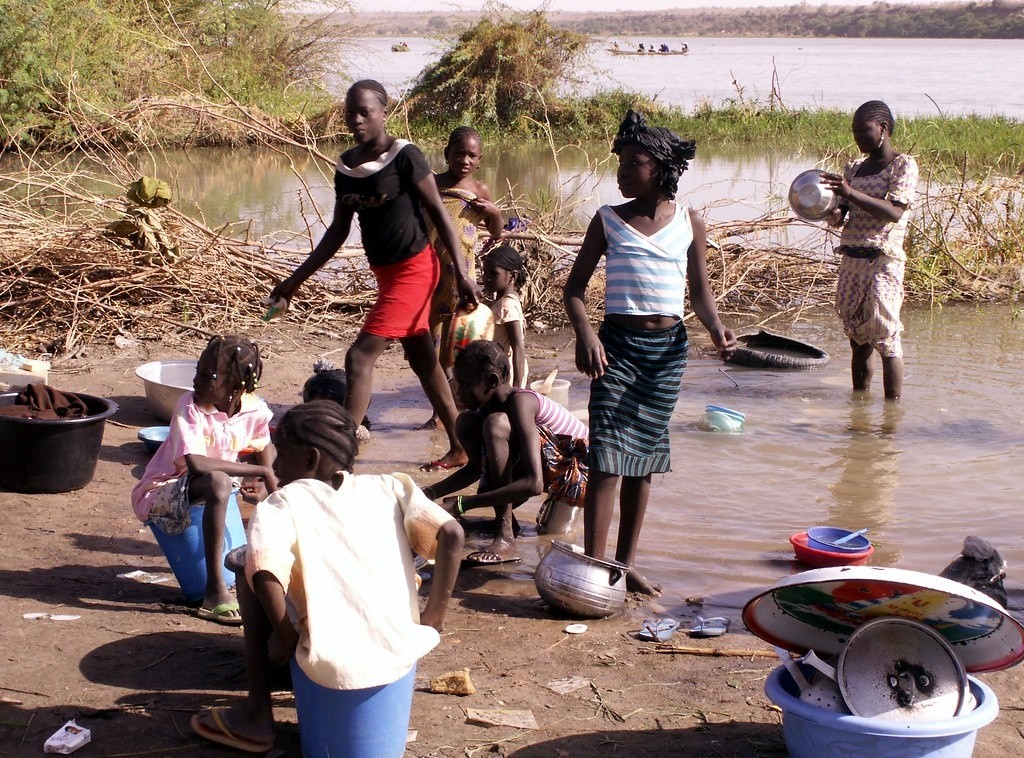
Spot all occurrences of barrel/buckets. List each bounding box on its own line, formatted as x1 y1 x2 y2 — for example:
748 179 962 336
287 648 416 758
530 378 570 411
143 488 249 603
0 392 119 492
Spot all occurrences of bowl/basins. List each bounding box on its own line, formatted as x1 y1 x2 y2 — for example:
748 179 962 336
765 659 1000 758
789 525 875 565
699 405 744 433
134 359 199 420
444 300 495 364
788 169 838 221
138 426 172 450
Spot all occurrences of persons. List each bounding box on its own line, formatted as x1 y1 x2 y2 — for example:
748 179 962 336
648 45 655 52
421 339 589 564
190 399 463 752
637 43 645 52
483 246 529 390
823 100 919 398
682 43 687 50
659 44 669 52
563 108 739 598
131 335 274 625
269 82 485 472
413 126 504 430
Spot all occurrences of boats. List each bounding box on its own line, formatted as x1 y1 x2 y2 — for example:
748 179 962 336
391 43 410 53
606 47 688 56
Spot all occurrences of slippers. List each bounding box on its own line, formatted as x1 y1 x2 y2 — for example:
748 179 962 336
639 619 680 641
420 460 451 472
199 602 243 622
467 550 521 562
688 616 730 636
191 706 272 752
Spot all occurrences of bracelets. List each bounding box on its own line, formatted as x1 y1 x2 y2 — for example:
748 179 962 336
430 488 435 501
457 495 465 514
